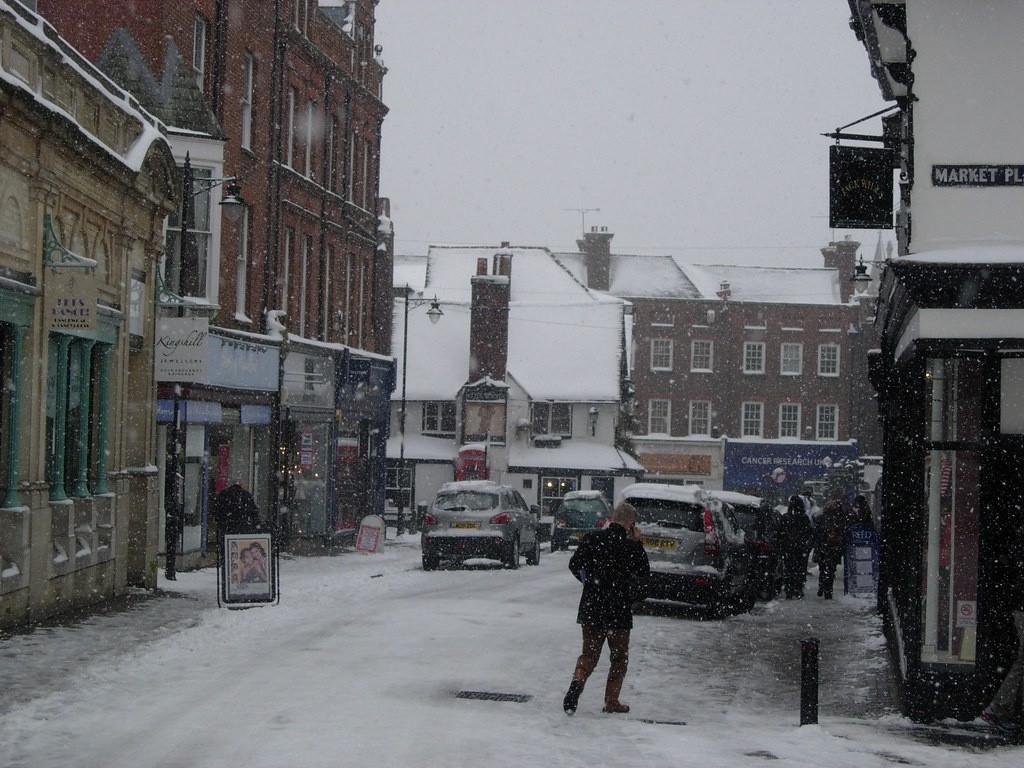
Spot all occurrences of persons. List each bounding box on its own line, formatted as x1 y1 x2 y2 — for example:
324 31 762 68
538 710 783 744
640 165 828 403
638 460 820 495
238 542 268 583
763 489 871 600
563 502 651 716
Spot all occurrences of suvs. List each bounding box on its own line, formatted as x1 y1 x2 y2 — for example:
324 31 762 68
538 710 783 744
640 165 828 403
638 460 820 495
551 490 614 553
421 481 542 571
614 483 784 619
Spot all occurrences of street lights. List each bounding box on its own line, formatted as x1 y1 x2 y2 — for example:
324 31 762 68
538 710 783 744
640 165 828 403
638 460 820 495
166 151 246 581
397 282 444 536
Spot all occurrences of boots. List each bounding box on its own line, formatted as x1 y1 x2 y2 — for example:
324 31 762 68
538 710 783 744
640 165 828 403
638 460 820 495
563 667 588 715
602 680 630 713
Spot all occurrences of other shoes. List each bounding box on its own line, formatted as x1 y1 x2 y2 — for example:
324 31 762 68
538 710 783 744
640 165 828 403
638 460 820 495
817 591 823 597
825 593 832 599
